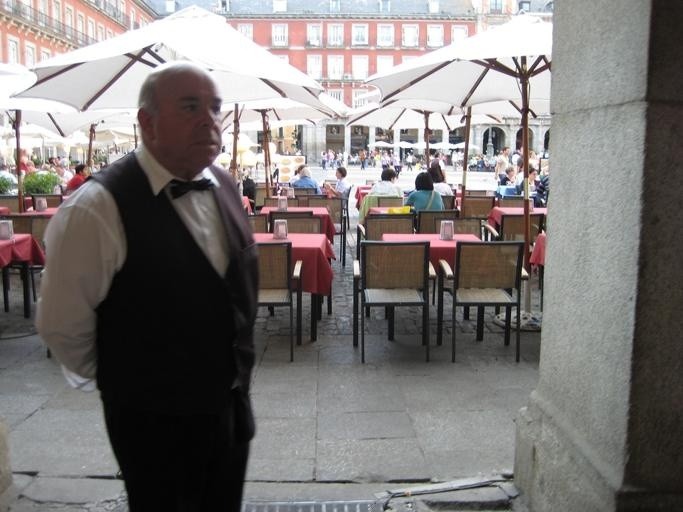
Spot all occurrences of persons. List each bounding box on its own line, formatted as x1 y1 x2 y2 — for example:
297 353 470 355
32 59 263 512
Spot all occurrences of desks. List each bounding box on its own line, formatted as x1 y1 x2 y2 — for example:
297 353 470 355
0 234 35 318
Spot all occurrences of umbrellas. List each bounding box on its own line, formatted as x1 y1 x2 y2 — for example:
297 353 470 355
361 11 555 311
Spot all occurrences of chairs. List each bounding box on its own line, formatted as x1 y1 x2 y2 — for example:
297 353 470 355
0 191 66 304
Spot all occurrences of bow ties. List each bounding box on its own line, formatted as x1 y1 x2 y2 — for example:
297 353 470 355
170 177 214 199
